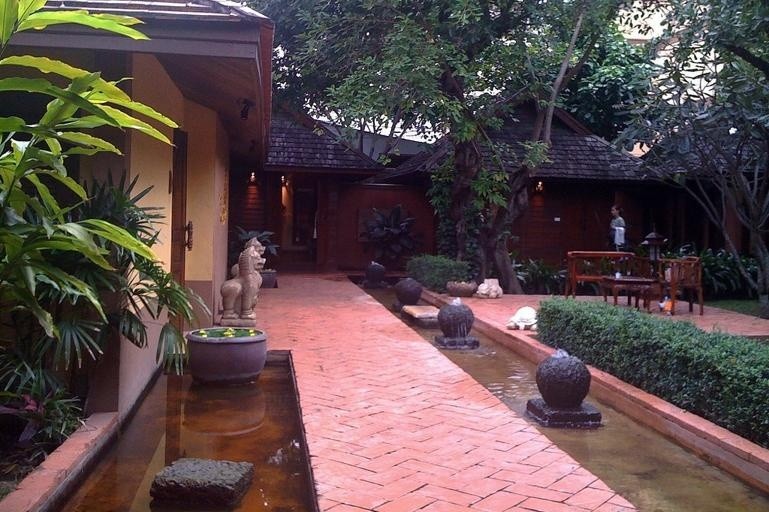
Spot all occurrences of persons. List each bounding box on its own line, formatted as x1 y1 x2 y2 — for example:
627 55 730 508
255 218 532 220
610 204 627 251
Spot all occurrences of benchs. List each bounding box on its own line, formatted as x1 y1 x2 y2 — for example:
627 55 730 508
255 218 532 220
567 246 639 301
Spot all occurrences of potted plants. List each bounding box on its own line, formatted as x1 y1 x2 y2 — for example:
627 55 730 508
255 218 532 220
233 224 279 288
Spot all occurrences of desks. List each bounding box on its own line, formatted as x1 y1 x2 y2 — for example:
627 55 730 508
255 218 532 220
605 274 649 309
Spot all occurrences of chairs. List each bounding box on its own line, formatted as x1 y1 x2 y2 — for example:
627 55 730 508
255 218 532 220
657 256 704 314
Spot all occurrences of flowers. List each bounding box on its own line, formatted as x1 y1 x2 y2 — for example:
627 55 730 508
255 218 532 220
192 328 261 338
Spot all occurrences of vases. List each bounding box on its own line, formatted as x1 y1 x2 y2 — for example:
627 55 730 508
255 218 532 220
181 382 267 438
186 328 267 387
